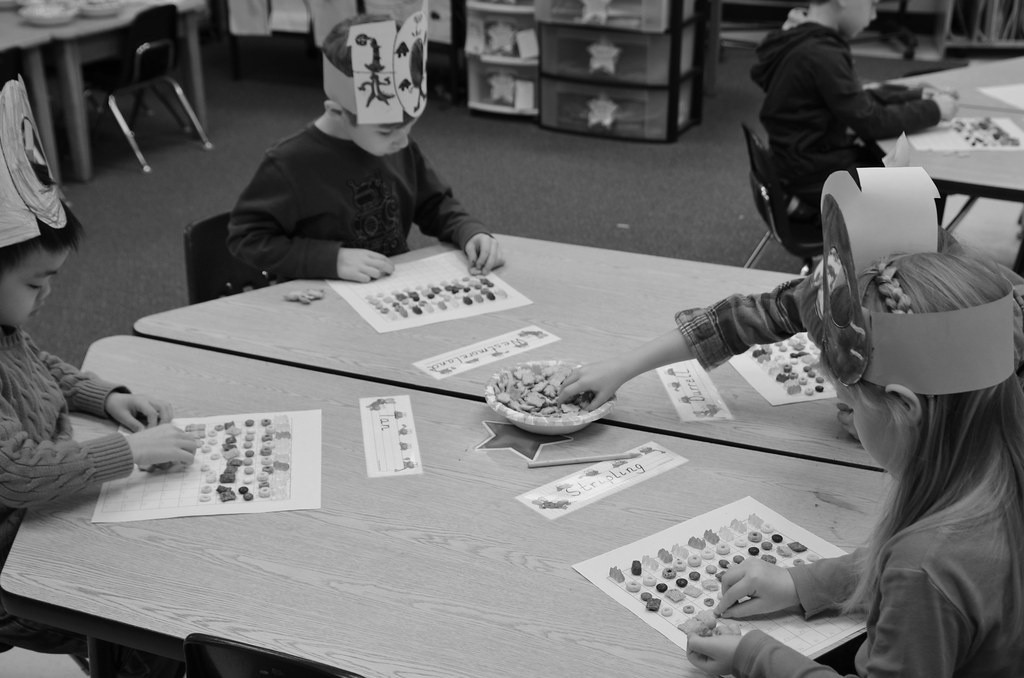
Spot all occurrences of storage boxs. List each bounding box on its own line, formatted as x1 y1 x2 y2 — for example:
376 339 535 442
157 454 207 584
464 0 708 141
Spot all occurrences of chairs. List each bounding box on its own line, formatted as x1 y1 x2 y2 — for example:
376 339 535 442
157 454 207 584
81 3 214 176
741 117 824 275
182 212 233 305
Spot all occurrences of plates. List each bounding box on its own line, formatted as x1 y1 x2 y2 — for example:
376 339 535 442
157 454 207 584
80 2 122 17
485 360 618 435
20 6 78 24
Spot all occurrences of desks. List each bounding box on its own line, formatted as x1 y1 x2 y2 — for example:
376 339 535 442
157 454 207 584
1 0 209 190
874 53 1024 282
0 233 896 678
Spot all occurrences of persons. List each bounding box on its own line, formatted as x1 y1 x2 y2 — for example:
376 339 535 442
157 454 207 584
554 224 1024 443
0 199 195 678
685 252 1024 678
747 0 966 259
219 16 506 297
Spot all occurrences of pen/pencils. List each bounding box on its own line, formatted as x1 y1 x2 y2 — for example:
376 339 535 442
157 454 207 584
528 452 642 468
